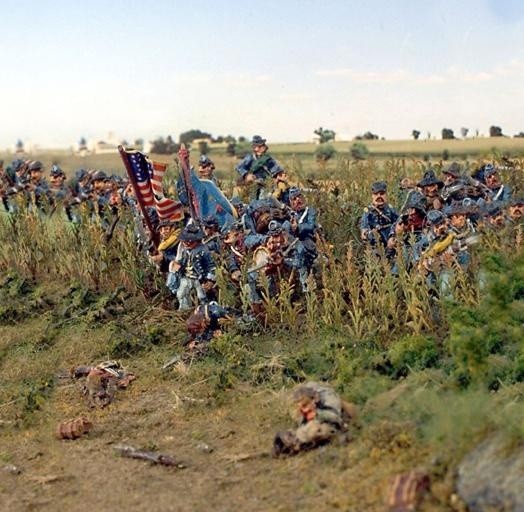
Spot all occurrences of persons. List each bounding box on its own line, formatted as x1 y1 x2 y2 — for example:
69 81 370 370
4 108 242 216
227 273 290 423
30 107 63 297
0 133 524 352
